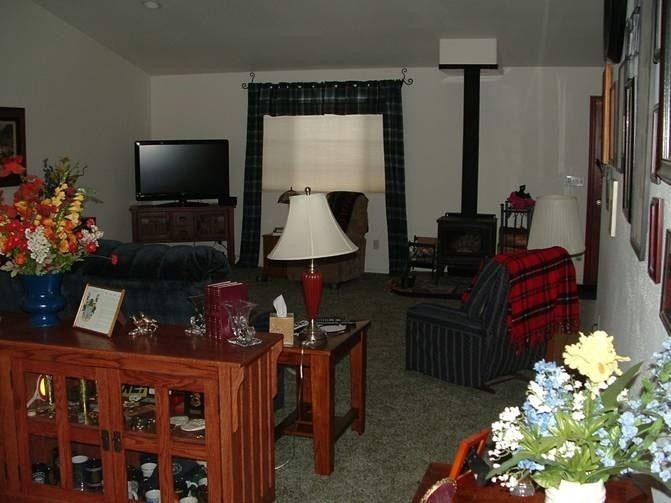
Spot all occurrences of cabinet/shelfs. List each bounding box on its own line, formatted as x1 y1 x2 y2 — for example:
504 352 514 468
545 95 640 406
0 312 284 502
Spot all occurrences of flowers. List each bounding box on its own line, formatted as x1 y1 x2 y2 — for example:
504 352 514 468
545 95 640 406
0 154 118 277
480 324 671 492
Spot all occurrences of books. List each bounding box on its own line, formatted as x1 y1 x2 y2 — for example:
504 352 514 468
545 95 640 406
272 226 287 236
316 310 361 327
205 279 245 339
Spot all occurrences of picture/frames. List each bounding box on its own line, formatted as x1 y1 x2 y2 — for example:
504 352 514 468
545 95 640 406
599 1 671 337
0 106 26 186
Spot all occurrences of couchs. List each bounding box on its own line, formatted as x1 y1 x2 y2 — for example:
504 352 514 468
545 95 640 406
0 239 233 331
284 193 370 289
402 247 568 394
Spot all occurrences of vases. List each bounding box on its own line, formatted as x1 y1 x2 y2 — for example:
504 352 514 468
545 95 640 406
19 271 66 327
651 480 671 502
544 475 609 501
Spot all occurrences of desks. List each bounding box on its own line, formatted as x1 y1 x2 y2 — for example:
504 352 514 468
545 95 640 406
455 460 671 502
128 203 234 266
271 320 372 476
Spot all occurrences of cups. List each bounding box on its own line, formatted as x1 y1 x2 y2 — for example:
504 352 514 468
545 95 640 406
226 300 261 346
30 447 207 502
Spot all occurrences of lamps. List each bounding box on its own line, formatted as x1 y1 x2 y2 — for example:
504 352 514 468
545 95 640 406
526 191 587 258
262 185 360 349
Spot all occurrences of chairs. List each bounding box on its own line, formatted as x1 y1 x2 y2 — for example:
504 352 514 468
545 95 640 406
422 423 490 502
401 234 439 289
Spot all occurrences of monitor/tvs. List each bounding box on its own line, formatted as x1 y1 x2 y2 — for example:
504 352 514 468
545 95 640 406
134 139 230 207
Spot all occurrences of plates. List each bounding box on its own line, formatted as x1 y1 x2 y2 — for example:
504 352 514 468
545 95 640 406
313 322 348 333
